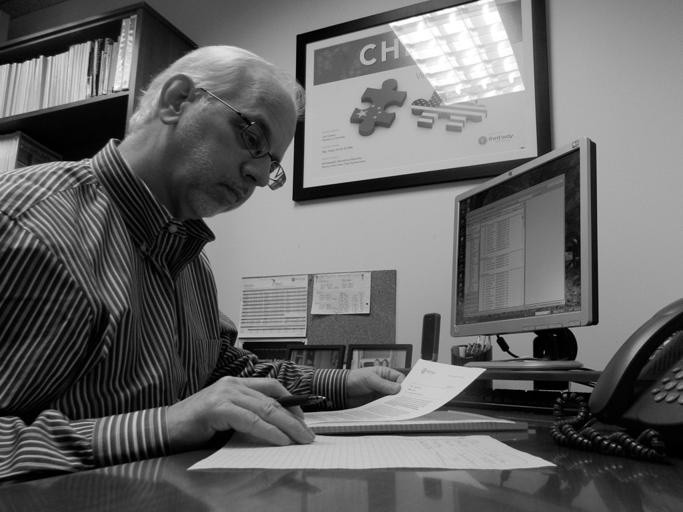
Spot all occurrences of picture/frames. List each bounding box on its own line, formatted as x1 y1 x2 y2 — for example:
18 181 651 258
284 343 346 377
346 342 414 377
292 0 555 203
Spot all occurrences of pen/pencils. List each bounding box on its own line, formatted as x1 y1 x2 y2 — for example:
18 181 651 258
465 336 491 357
277 395 326 408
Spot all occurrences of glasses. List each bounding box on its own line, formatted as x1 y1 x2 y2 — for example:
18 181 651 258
194 87 286 191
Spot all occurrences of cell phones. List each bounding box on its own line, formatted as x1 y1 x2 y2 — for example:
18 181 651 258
421 313 440 361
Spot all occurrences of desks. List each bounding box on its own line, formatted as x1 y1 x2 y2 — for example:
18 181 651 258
1 428 681 510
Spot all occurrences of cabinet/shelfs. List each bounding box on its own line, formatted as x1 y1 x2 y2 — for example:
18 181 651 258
0 0 198 139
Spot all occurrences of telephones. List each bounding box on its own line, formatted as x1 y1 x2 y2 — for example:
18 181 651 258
588 296 683 449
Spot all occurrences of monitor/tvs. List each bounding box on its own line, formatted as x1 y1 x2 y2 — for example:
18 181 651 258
450 136 599 369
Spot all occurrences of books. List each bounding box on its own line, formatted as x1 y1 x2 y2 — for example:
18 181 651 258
411 430 531 441
304 409 530 436
0 11 140 173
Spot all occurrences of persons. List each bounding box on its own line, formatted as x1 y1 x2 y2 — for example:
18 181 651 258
372 358 380 367
0 43 406 491
97 457 305 510
381 359 388 367
